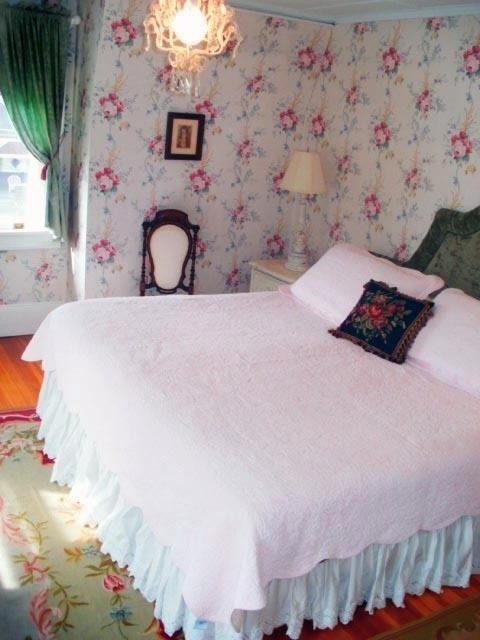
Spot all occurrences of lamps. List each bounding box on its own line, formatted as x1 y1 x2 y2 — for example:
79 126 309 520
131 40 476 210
135 0 245 95
278 150 328 271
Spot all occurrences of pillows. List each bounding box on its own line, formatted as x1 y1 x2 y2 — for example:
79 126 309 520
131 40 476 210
289 241 479 423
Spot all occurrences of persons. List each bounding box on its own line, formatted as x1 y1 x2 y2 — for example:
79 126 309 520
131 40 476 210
176 126 191 148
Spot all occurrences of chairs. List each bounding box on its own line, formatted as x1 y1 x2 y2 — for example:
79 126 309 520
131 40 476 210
141 210 201 296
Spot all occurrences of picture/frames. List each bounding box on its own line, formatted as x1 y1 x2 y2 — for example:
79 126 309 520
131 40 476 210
163 111 206 161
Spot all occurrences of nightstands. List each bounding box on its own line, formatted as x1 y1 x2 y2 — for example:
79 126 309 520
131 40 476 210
249 258 310 293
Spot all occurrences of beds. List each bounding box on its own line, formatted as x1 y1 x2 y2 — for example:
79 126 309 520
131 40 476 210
20 207 479 639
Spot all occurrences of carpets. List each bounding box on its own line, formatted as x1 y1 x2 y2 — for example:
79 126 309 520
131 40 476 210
0 411 480 640
